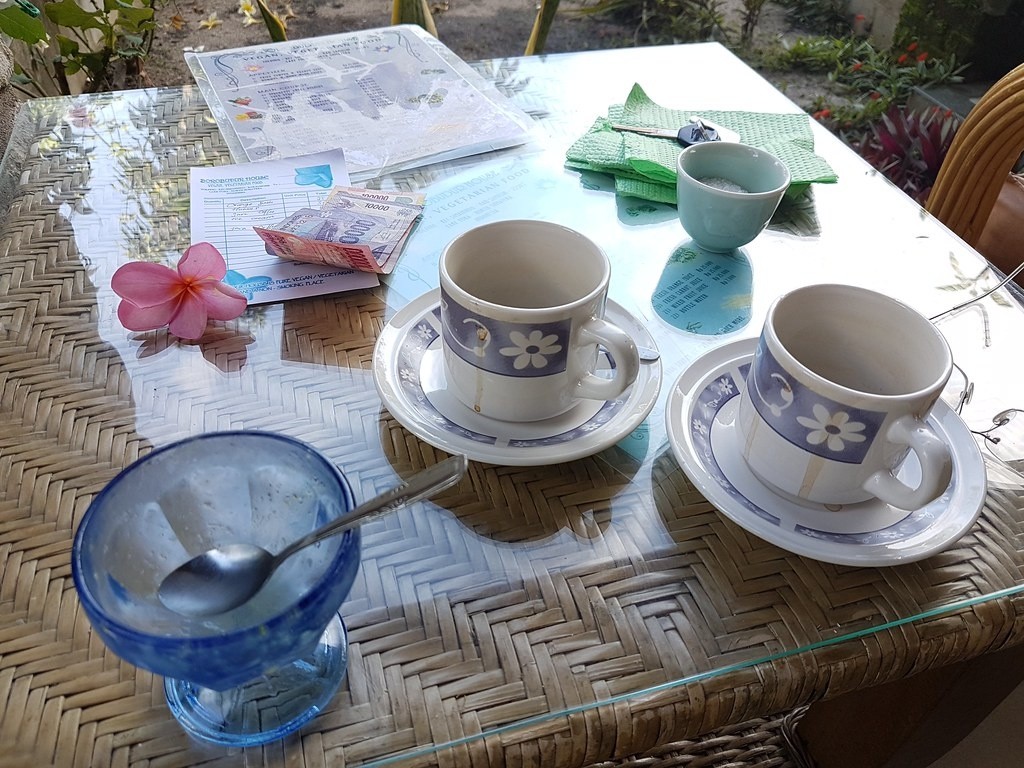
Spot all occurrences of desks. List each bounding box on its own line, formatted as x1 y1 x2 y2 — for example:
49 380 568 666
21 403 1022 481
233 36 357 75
1 42 1024 768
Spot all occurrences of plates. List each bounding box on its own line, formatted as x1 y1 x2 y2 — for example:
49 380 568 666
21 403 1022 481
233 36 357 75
374 287 662 465
664 338 987 565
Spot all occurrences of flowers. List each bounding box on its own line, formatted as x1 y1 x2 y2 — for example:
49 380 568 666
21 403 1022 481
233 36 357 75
111 246 249 344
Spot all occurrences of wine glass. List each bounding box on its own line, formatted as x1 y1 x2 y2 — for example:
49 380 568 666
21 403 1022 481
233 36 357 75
71 432 347 746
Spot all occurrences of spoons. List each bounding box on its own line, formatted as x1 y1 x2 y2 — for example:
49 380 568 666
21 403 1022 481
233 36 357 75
157 454 467 615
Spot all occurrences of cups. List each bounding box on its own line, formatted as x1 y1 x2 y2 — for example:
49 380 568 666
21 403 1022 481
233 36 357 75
439 220 639 423
677 141 790 254
737 286 952 511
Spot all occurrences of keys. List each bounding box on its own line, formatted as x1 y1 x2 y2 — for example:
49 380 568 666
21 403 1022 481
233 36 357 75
612 121 720 148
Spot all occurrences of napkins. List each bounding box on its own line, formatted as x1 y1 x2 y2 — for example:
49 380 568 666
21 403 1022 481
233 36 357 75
560 81 842 201
